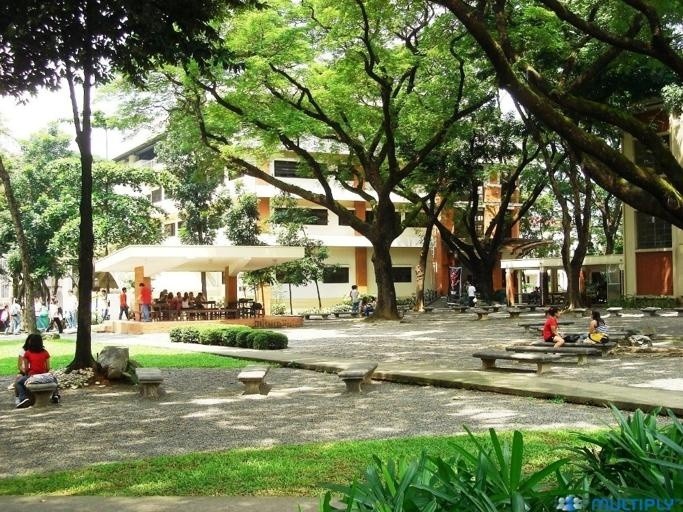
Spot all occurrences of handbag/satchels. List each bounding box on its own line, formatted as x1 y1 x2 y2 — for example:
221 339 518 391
601 336 609 343
564 334 580 343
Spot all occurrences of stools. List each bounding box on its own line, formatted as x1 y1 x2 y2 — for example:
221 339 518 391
24 381 58 407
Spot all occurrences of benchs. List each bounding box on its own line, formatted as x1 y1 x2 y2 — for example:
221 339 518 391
517 320 575 331
572 305 683 319
422 307 434 313
453 304 537 318
472 340 616 374
135 366 164 399
303 312 359 320
238 365 271 391
338 361 377 391
127 307 237 321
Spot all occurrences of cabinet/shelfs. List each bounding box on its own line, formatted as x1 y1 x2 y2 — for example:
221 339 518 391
236 302 264 318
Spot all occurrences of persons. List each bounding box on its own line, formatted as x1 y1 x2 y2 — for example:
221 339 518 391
0 295 64 333
543 306 565 347
350 285 377 317
463 281 478 307
119 282 206 321
14 333 61 408
575 310 609 345
97 289 110 324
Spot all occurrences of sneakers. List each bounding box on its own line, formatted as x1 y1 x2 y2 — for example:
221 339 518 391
52 396 60 403
15 396 30 408
